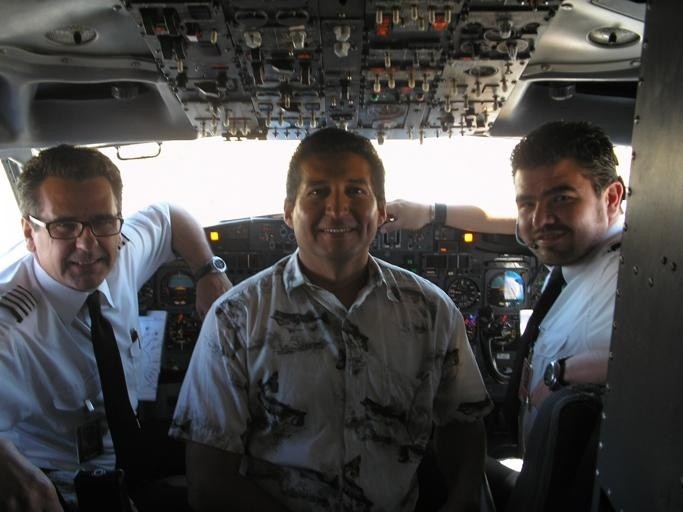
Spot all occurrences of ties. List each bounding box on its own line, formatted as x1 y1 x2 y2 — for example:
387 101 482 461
503 264 565 444
86 290 153 507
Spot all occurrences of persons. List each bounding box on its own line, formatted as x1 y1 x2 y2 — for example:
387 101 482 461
374 121 624 510
168 124 490 510
0 140 238 510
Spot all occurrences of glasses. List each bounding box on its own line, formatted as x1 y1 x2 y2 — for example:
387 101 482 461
24 212 125 241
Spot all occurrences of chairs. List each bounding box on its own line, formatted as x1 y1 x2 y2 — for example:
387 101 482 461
484 381 604 511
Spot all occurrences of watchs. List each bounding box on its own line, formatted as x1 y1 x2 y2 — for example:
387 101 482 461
193 254 228 281
541 352 574 391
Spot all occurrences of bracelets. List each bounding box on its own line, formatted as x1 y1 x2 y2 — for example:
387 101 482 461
428 202 447 227
